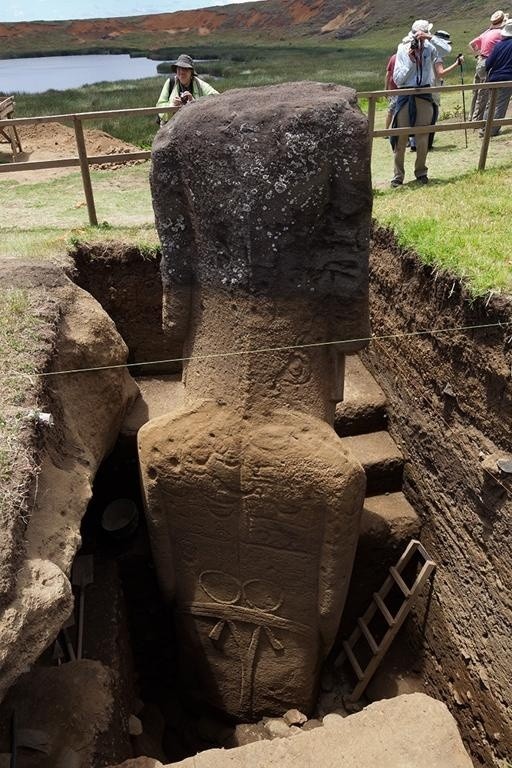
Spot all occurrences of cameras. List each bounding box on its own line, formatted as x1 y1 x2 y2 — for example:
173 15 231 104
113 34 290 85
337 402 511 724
179 92 188 102
410 37 419 50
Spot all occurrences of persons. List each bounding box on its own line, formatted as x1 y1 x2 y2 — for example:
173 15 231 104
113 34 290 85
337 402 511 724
467 9 509 127
154 53 224 126
474 20 512 140
390 18 438 188
424 29 465 151
383 53 400 139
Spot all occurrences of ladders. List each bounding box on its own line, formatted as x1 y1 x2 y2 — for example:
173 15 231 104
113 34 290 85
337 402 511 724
335 539 436 702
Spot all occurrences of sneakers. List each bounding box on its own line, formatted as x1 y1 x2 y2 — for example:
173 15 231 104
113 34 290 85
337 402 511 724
416 175 429 184
390 176 404 188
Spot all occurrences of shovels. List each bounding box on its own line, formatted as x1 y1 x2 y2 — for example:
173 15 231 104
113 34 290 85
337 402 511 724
73 554 95 661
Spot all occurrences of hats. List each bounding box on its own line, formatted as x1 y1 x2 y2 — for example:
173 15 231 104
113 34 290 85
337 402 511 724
407 19 434 38
434 30 452 45
489 10 512 37
170 53 198 78
